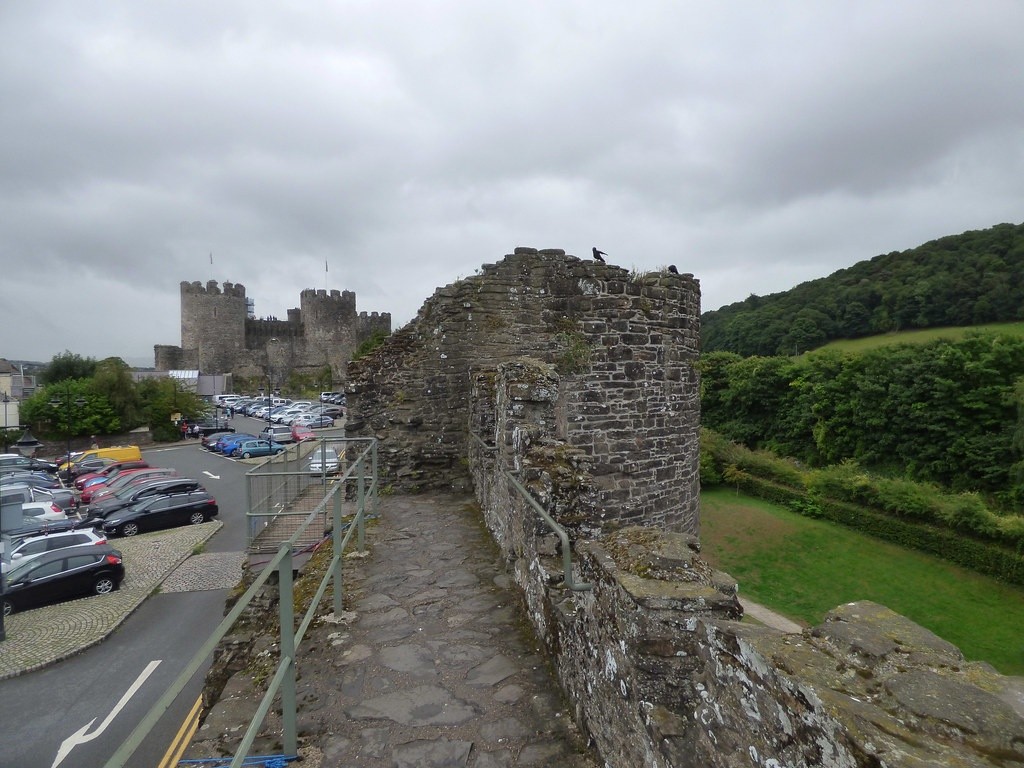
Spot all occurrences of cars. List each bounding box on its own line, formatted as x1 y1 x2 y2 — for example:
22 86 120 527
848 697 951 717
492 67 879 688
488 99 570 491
200 392 347 457
0 443 201 576
0 544 125 618
291 424 317 442
308 447 340 476
236 439 287 460
101 486 218 538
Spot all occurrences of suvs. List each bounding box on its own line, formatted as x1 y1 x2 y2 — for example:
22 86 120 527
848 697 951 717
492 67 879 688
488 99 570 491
258 424 293 445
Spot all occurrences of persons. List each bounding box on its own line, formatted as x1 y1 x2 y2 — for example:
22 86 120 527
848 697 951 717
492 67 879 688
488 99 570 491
181 404 247 440
90 442 100 449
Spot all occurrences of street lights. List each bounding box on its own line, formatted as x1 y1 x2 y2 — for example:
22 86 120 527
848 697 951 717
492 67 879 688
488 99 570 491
66 383 72 484
265 375 272 456
314 383 322 428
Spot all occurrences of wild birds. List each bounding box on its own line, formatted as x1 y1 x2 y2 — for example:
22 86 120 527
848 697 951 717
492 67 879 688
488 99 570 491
592 247 607 262
667 264 679 274
